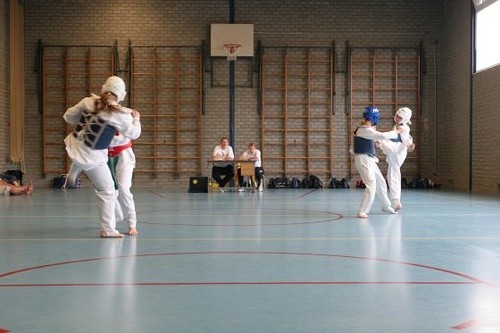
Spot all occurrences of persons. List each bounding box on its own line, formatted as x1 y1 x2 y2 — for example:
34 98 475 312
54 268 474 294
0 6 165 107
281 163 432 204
377 107 415 211
351 106 399 217
61 76 141 237
238 143 262 191
210 137 235 194
0 178 32 196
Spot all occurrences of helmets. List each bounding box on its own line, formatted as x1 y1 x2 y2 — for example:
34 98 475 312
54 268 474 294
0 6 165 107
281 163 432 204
101 75 126 103
394 107 412 124
363 105 380 125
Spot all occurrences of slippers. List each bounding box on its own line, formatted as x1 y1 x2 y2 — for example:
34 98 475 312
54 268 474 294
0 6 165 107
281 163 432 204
100 230 125 238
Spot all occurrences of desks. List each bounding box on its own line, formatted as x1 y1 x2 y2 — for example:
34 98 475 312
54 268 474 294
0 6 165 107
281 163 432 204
207 160 257 193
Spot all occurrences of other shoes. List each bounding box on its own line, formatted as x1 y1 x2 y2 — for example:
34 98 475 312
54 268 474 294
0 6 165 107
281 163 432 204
258 188 263 191
238 188 244 191
218 189 224 192
223 187 226 191
